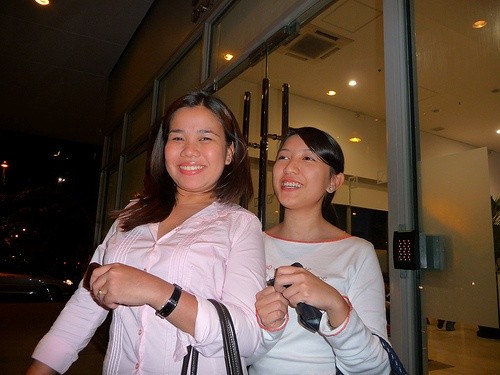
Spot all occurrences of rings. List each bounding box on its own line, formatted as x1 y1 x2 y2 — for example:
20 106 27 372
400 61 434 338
98 290 107 295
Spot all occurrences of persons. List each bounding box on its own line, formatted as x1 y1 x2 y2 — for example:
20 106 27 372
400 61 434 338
247 127 394 375
25 91 265 375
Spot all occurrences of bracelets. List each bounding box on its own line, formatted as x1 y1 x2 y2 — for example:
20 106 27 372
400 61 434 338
155 283 182 320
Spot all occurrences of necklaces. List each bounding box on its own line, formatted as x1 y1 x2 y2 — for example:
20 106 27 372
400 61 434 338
169 197 216 230
